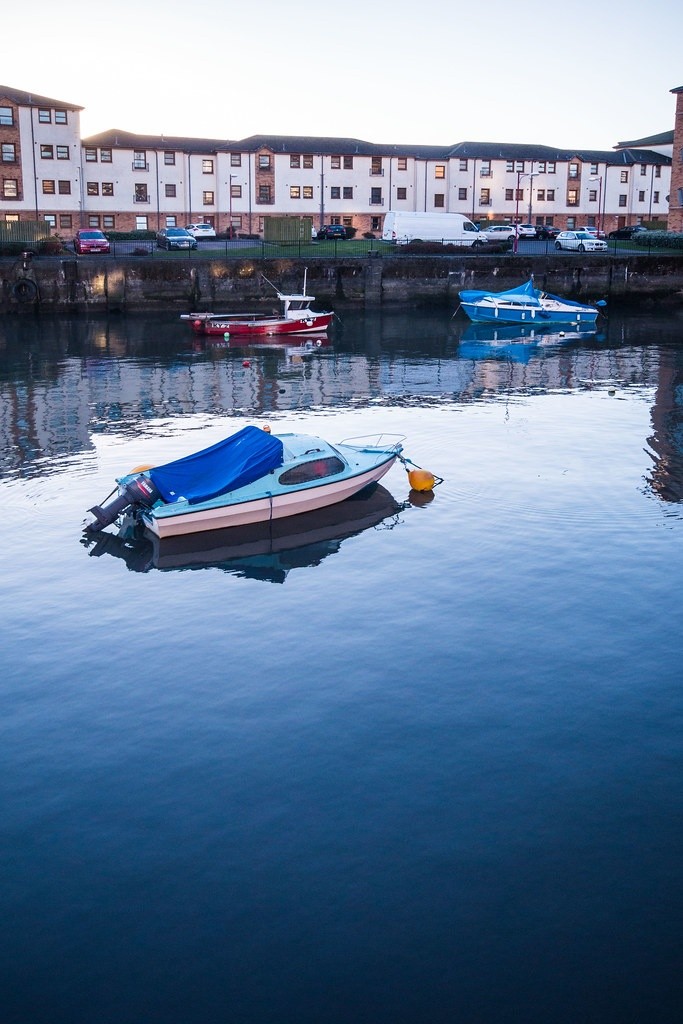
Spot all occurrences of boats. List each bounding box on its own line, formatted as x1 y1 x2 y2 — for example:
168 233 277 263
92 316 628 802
81 424 445 540
458 273 607 324
179 267 334 336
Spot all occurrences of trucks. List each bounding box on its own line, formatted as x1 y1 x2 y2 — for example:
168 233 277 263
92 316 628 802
382 211 489 247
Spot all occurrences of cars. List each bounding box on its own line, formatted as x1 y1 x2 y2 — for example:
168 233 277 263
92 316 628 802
156 226 198 250
534 225 561 241
480 225 520 244
74 229 110 254
609 225 647 240
554 231 608 252
311 225 317 240
317 224 346 240
509 224 537 240
185 224 216 241
570 227 605 239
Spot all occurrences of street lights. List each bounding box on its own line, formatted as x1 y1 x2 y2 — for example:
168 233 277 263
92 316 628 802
588 176 603 239
513 171 540 252
